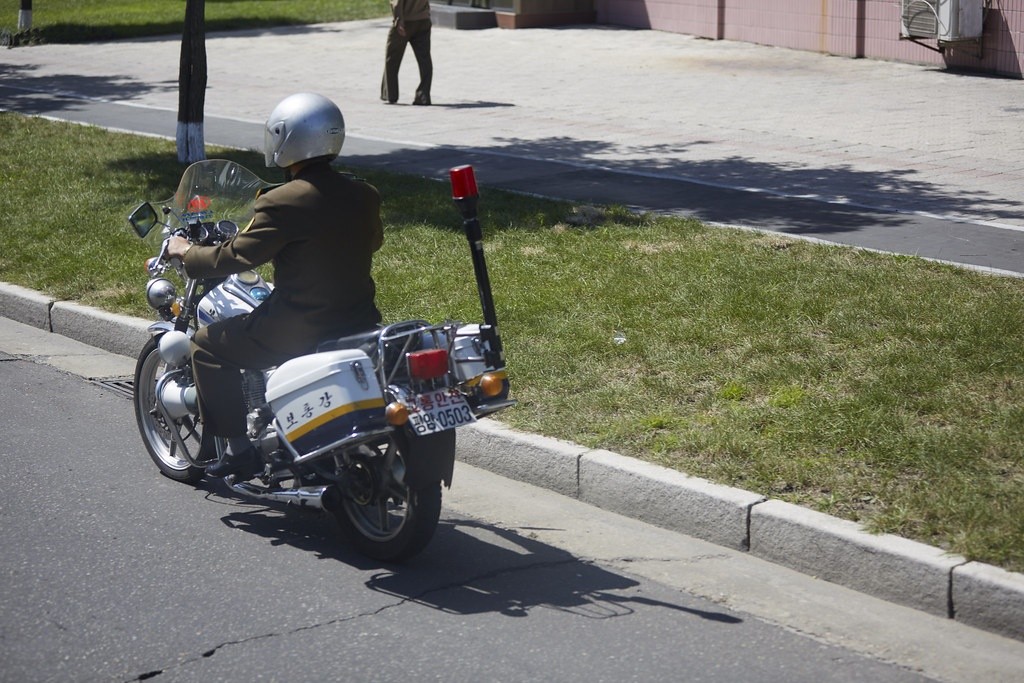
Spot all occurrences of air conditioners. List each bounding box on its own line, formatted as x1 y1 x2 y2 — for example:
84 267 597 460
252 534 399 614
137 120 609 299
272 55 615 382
901 0 984 42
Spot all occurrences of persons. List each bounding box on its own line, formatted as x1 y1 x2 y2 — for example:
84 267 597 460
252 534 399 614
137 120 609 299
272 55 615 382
380 0 433 106
162 91 385 480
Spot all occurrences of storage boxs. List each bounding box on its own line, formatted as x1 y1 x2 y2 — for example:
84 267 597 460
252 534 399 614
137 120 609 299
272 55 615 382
422 324 510 408
264 348 386 457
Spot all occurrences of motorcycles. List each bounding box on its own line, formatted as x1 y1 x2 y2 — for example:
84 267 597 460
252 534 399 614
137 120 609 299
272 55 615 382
129 159 521 560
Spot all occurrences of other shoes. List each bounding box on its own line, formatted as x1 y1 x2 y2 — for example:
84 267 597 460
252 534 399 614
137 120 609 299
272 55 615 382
413 100 430 105
386 100 396 105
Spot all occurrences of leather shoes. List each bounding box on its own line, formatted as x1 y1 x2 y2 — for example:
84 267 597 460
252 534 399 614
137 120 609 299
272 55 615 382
204 442 266 480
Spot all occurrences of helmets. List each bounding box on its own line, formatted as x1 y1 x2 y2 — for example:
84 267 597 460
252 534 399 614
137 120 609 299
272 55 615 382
267 92 345 169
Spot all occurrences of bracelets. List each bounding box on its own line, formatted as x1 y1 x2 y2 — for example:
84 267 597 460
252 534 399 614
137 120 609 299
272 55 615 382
183 245 190 257
